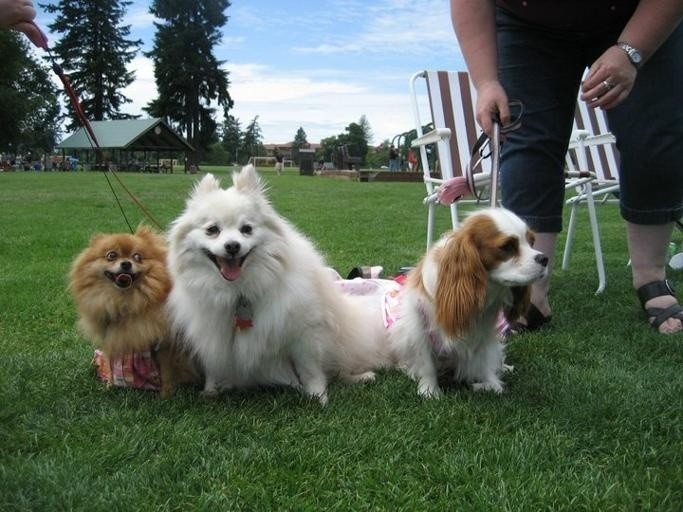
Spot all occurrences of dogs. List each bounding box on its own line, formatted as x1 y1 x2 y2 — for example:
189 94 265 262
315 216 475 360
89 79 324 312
388 201 550 402
161 163 386 408
66 220 191 402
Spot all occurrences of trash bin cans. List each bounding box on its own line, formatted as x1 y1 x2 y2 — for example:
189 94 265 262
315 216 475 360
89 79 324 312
91 160 110 172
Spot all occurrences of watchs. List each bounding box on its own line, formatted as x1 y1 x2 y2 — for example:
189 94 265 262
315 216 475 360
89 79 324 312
614 42 645 73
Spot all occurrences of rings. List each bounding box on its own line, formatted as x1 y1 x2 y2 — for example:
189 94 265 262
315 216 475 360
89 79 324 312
602 81 611 91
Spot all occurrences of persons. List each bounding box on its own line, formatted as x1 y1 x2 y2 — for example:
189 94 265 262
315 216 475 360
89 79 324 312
0 147 199 174
0 0 48 50
387 143 398 174
442 0 683 342
272 146 285 177
405 144 418 170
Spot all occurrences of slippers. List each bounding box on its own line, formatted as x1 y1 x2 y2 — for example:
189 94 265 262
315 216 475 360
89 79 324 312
498 298 551 337
637 278 682 336
